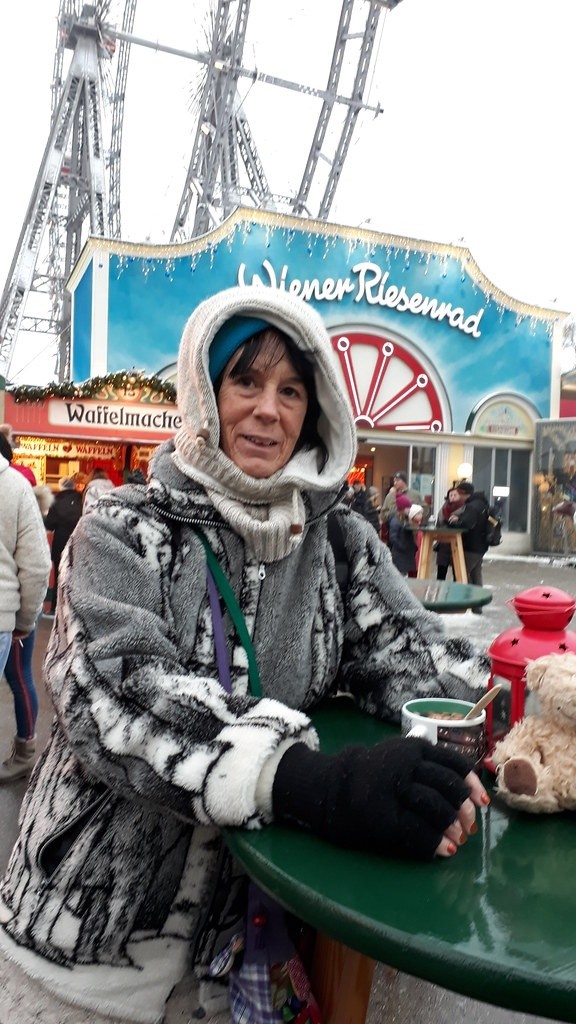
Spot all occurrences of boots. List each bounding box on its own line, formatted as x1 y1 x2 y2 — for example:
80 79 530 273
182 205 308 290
0 732 37 784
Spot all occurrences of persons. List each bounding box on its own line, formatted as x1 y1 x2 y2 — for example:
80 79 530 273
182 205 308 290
431 483 489 614
0 285 534 1024
43 468 116 619
344 471 428 578
0 424 52 787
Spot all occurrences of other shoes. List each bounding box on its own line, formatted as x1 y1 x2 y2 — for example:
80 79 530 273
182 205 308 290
41 608 54 619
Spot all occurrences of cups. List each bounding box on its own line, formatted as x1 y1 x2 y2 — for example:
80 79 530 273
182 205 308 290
401 697 487 770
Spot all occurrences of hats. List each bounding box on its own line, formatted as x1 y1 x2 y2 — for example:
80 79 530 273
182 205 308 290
408 504 424 520
394 471 408 485
59 476 75 491
172 265 357 534
369 486 380 496
396 492 412 511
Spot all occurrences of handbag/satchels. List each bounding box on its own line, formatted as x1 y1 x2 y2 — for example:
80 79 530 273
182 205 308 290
210 881 325 1024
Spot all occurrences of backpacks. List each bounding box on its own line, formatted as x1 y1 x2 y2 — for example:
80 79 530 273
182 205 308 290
481 499 504 546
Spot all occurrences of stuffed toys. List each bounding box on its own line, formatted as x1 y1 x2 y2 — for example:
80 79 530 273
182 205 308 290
493 651 576 813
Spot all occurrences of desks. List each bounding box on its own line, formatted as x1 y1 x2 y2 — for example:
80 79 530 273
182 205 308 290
227 696 576 1024
401 577 495 615
409 525 471 583
